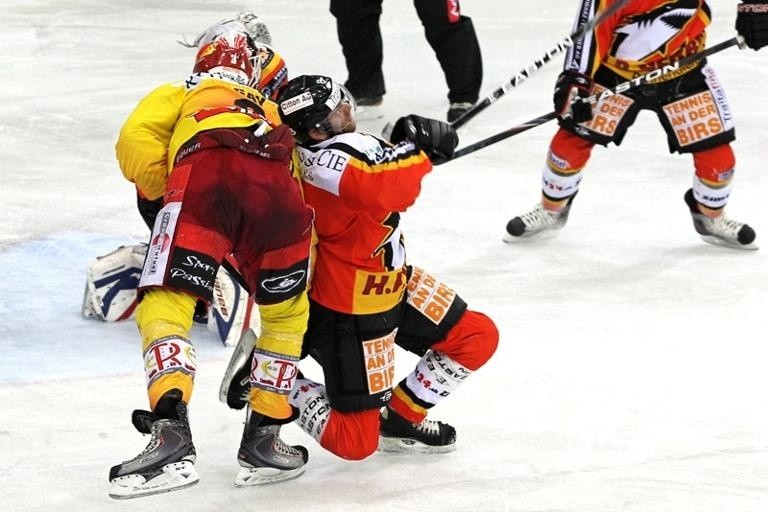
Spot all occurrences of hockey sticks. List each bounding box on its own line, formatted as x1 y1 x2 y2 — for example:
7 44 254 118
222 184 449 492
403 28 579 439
382 36 743 165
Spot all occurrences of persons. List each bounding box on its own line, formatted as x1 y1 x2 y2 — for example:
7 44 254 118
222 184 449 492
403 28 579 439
325 0 482 123
501 0 768 253
102 9 315 497
80 44 288 344
217 74 498 462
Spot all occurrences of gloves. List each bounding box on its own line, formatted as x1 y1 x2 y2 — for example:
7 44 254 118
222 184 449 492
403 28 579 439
389 114 458 162
553 70 591 129
735 0 766 49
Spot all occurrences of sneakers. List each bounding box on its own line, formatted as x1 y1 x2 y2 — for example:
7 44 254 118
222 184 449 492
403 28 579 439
448 101 474 121
226 345 255 410
684 188 754 243
505 191 578 236
378 407 455 446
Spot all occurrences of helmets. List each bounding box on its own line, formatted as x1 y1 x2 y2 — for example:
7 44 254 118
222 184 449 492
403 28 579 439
276 74 344 144
193 42 287 102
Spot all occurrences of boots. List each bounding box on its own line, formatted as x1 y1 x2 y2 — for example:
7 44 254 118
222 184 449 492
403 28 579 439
238 405 307 470
110 387 195 482
